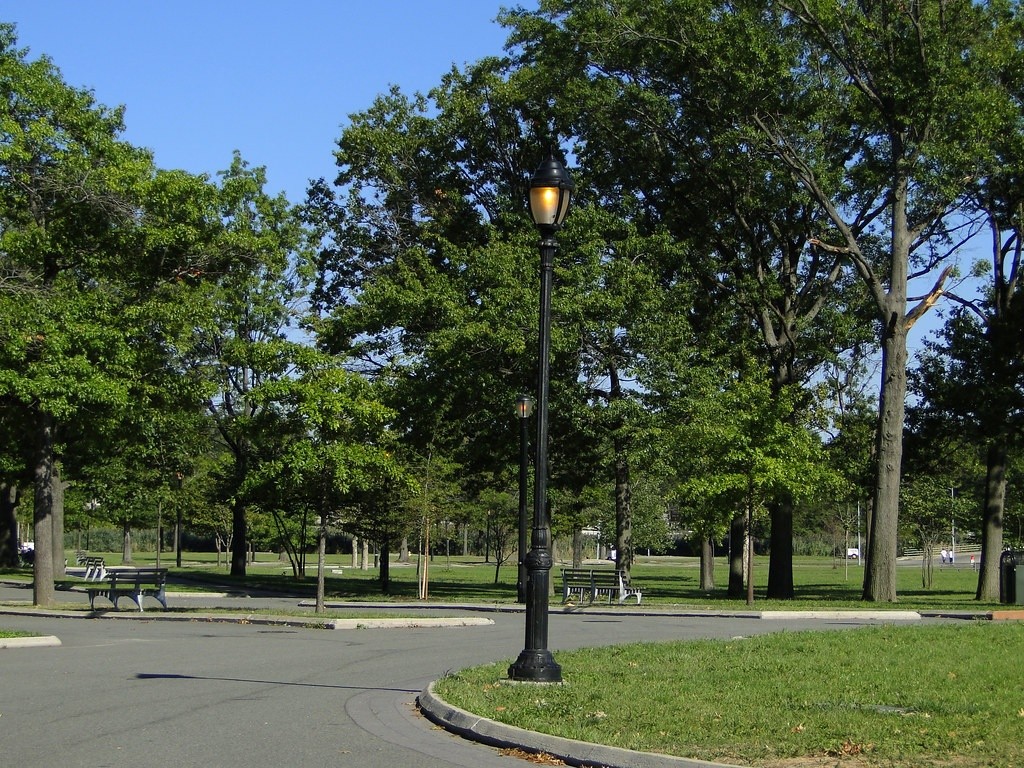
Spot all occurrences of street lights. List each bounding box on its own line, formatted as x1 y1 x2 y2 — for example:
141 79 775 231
512 394 534 604
507 153 577 681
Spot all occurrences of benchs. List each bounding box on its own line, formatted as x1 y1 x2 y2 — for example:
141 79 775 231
590 569 642 604
559 568 595 604
83 556 118 583
85 568 170 612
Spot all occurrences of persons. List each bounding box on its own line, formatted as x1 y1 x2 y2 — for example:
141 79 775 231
940 548 947 563
948 549 953 563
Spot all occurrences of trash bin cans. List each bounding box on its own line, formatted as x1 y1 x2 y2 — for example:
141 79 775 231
999 550 1024 606
